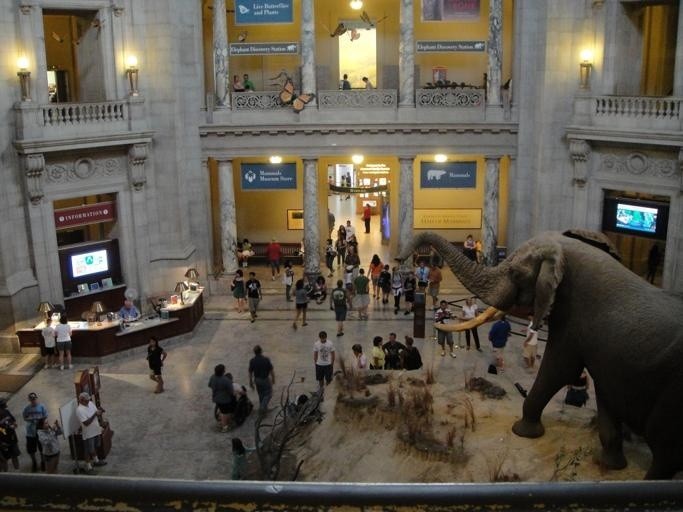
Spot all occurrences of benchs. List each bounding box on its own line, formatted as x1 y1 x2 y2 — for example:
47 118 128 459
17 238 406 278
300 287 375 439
237 241 302 265
413 241 465 265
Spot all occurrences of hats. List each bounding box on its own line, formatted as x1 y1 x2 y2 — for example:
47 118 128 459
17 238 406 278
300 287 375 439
78 391 91 401
28 392 37 401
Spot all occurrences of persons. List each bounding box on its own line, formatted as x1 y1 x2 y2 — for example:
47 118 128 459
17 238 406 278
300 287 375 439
247 344 276 419
368 254 443 316
206 363 236 432
39 317 58 369
0 393 107 474
324 203 373 279
339 74 351 90
434 300 457 358
314 331 413 392
463 235 477 261
461 297 483 353
230 436 256 479
361 77 373 90
232 74 245 92
223 372 252 415
144 334 167 394
116 298 141 324
232 239 263 324
52 314 75 371
487 311 511 377
286 261 370 339
241 74 256 92
327 171 351 202
266 237 283 280
520 314 537 369
564 371 590 408
244 85 253 94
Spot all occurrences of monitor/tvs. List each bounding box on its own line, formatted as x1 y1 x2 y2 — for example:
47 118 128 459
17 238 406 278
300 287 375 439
69 247 110 280
614 203 659 235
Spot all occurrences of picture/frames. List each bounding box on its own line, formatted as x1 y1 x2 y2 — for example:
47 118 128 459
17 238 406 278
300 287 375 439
286 208 304 231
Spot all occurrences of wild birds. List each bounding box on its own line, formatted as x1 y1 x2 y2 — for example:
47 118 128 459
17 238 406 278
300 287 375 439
361 11 388 31
350 30 360 42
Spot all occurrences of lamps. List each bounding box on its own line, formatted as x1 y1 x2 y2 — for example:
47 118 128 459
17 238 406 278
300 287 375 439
577 48 593 90
90 301 107 322
174 268 199 305
36 301 55 324
17 58 33 102
124 55 145 97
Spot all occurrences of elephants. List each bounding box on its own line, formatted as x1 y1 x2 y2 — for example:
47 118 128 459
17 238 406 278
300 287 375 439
393 227 683 481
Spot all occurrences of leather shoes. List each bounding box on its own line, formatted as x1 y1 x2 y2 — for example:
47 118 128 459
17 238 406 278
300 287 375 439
93 461 107 466
336 333 343 336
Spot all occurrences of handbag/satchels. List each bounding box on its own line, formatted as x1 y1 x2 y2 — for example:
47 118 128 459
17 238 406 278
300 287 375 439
230 276 237 290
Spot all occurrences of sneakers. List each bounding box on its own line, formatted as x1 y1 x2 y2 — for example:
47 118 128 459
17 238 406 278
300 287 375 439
222 424 232 433
441 350 445 356
44 363 71 370
450 351 456 358
31 462 36 472
40 461 46 471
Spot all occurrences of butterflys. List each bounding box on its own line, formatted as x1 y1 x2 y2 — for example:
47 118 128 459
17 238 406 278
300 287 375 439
279 77 315 113
